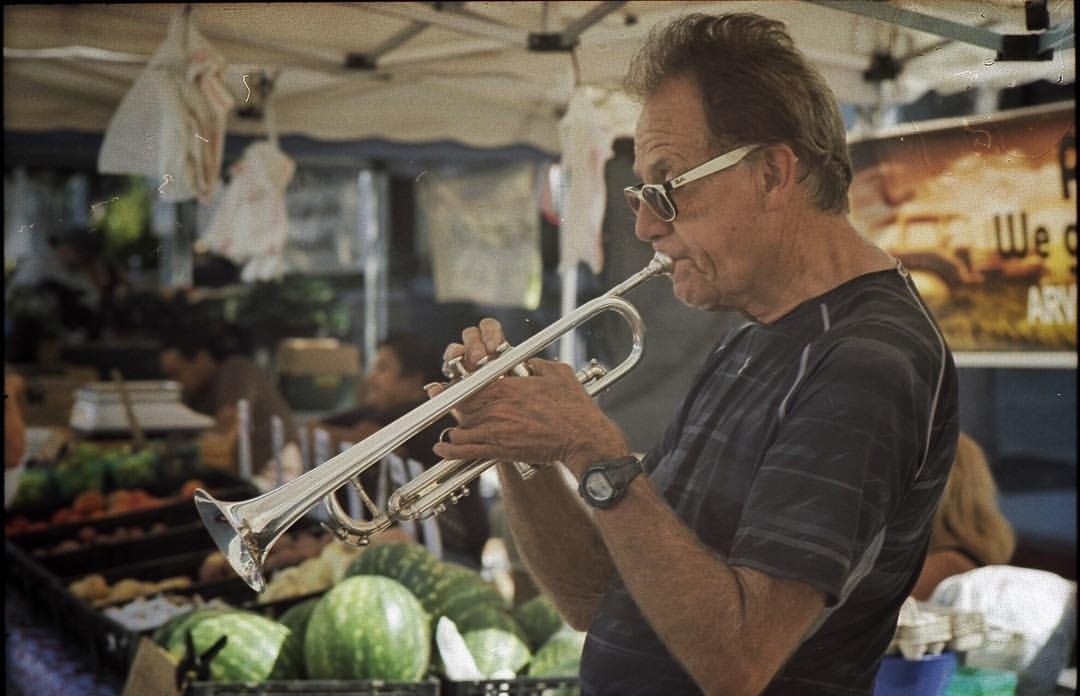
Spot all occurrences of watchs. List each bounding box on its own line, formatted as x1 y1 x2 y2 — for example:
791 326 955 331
577 456 643 509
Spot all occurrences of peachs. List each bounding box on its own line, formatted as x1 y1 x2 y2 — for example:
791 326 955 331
4 479 418 604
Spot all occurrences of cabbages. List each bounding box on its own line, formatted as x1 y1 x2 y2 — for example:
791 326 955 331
17 436 196 512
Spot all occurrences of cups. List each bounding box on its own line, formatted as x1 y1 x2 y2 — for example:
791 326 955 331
944 666 1018 696
874 650 958 696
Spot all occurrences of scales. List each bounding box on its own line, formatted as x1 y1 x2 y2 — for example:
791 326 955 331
69 378 213 439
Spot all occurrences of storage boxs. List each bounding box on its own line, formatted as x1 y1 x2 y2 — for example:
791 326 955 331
1 463 580 696
277 336 358 375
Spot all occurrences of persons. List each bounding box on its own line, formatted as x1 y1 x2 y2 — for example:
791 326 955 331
909 433 1015 601
427 11 961 696
4 282 491 570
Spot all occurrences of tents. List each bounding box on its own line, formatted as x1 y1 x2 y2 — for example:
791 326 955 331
2 0 1078 544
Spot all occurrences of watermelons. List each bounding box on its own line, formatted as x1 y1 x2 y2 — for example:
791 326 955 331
152 541 588 696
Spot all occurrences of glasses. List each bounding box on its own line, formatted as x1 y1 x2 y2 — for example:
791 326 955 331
623 143 800 221
